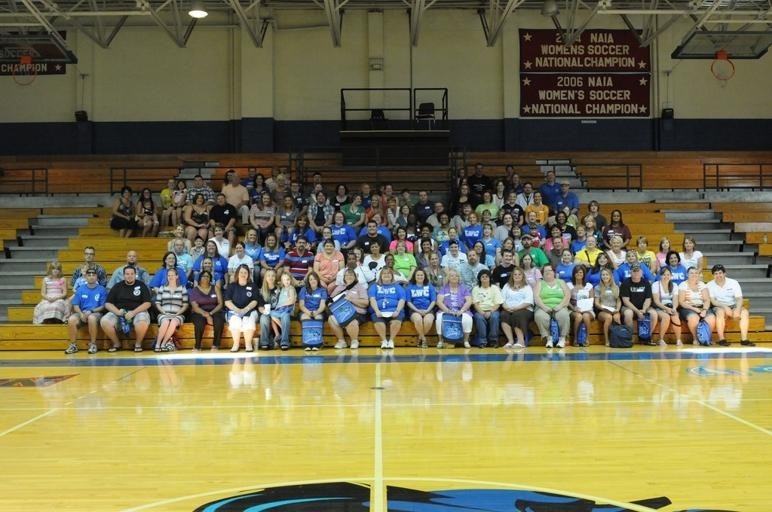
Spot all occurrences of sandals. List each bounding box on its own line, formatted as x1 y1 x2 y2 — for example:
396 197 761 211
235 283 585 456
154 344 169 352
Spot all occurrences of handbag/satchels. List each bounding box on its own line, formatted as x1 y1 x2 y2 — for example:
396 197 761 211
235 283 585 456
637 312 651 341
442 312 464 341
577 323 587 344
608 322 633 348
550 318 559 344
328 293 358 326
696 320 712 346
301 319 324 347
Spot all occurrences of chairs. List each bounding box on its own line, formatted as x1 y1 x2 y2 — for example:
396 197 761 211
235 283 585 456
369 109 384 130
415 103 436 130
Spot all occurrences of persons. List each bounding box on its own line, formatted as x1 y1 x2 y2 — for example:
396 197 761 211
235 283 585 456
30 163 755 351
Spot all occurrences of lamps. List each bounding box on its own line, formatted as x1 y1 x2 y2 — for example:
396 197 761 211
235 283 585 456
541 0 559 18
187 0 208 19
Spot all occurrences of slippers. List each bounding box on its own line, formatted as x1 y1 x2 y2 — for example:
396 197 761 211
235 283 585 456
108 346 122 352
134 346 142 352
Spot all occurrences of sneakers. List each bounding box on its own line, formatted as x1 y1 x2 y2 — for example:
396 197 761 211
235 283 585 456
350 339 360 349
643 340 754 346
462 339 471 349
261 345 269 350
230 345 240 352
417 336 429 348
334 341 348 349
437 338 444 349
305 347 320 351
281 345 289 350
245 345 253 352
65 344 78 354
211 345 218 351
273 335 281 342
381 340 394 349
480 343 486 348
490 343 526 349
546 336 566 349
572 341 590 347
88 343 98 354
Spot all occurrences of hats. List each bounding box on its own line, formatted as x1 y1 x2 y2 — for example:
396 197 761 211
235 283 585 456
521 233 532 240
630 263 641 270
